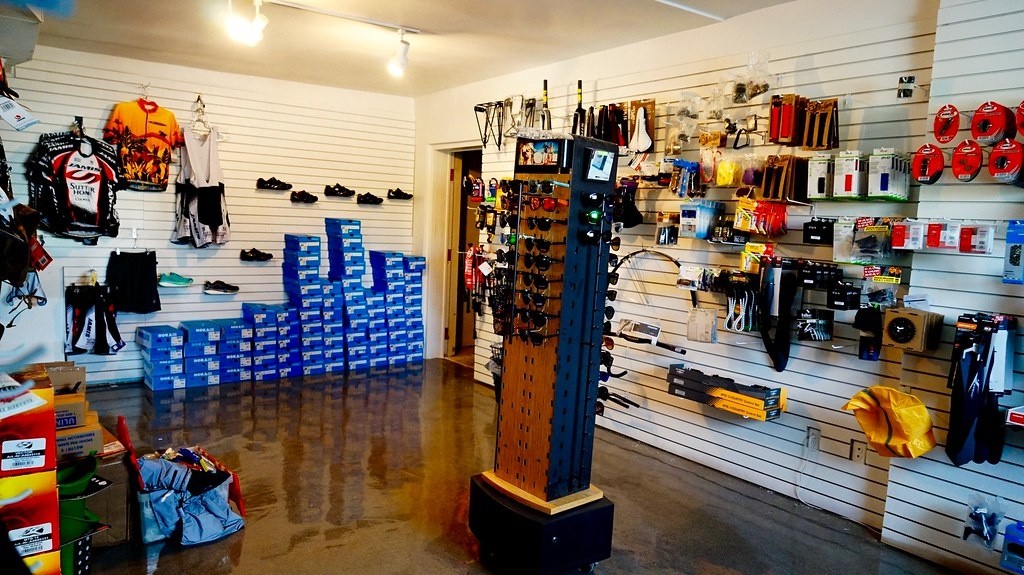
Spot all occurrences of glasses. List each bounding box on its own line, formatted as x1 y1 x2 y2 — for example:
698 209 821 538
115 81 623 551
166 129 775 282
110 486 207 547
577 186 624 417
488 179 569 346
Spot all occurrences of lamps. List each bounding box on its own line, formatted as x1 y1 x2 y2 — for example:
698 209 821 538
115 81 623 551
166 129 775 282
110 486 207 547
243 14 269 47
388 40 410 77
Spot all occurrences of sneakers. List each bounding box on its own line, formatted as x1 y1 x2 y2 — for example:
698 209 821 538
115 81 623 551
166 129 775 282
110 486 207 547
158 272 193 287
387 187 413 201
323 182 355 198
203 280 239 295
254 176 292 190
289 189 319 203
356 192 384 204
239 247 274 262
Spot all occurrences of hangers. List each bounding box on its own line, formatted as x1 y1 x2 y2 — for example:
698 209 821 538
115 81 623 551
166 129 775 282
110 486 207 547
113 231 156 255
66 269 110 293
182 108 232 141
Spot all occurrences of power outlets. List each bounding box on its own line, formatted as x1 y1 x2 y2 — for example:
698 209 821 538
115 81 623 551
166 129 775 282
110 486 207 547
808 428 820 450
852 441 867 465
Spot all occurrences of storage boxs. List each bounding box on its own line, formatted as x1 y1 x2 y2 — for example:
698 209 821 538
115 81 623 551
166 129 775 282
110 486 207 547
135 325 184 349
678 198 716 239
220 366 251 384
141 345 182 363
215 338 251 355
183 341 216 358
670 364 781 400
325 217 361 234
668 384 781 422
666 372 778 410
145 371 186 392
179 320 220 344
143 357 182 378
185 370 219 388
210 318 253 341
220 353 252 368
242 234 426 380
184 356 220 374
0 361 131 575
807 148 912 202
